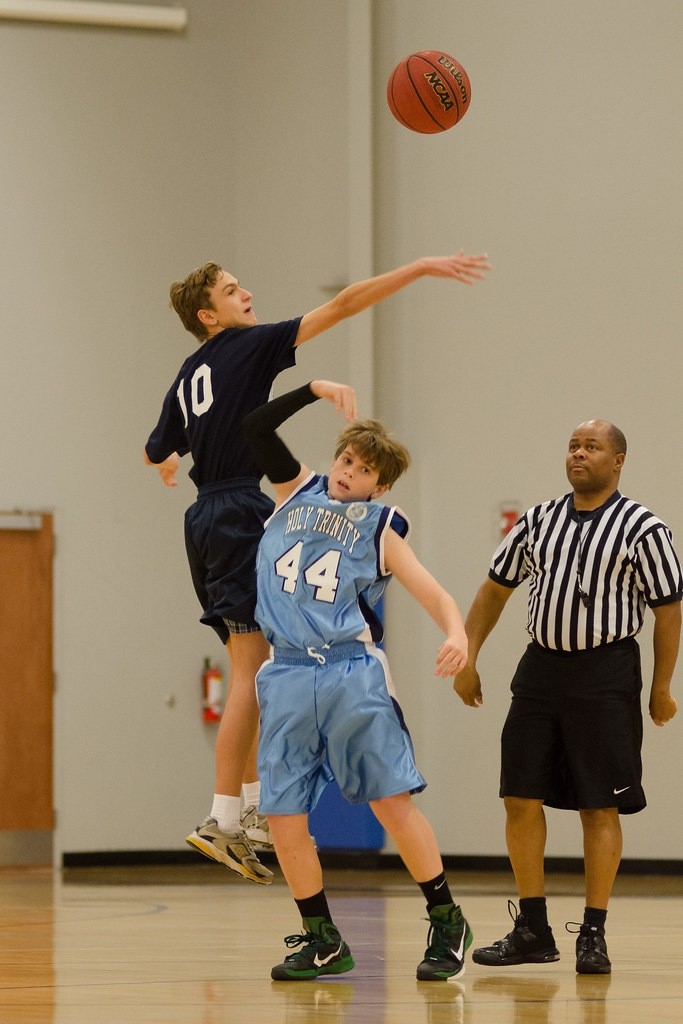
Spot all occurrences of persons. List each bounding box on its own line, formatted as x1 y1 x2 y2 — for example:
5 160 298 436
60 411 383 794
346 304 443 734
141 249 492 886
237 377 475 982
452 418 683 975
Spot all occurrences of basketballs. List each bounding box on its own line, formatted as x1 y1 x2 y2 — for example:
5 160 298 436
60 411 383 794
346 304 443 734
386 49 473 135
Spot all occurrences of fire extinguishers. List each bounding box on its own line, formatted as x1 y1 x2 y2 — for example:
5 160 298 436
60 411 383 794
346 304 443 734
204 657 224 724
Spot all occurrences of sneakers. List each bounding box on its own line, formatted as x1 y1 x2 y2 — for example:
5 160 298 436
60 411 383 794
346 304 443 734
414 901 473 982
240 805 318 855
185 816 275 886
565 921 612 974
272 916 356 979
467 900 560 967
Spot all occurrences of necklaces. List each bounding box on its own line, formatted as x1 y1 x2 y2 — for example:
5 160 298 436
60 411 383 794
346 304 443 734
572 498 599 607
